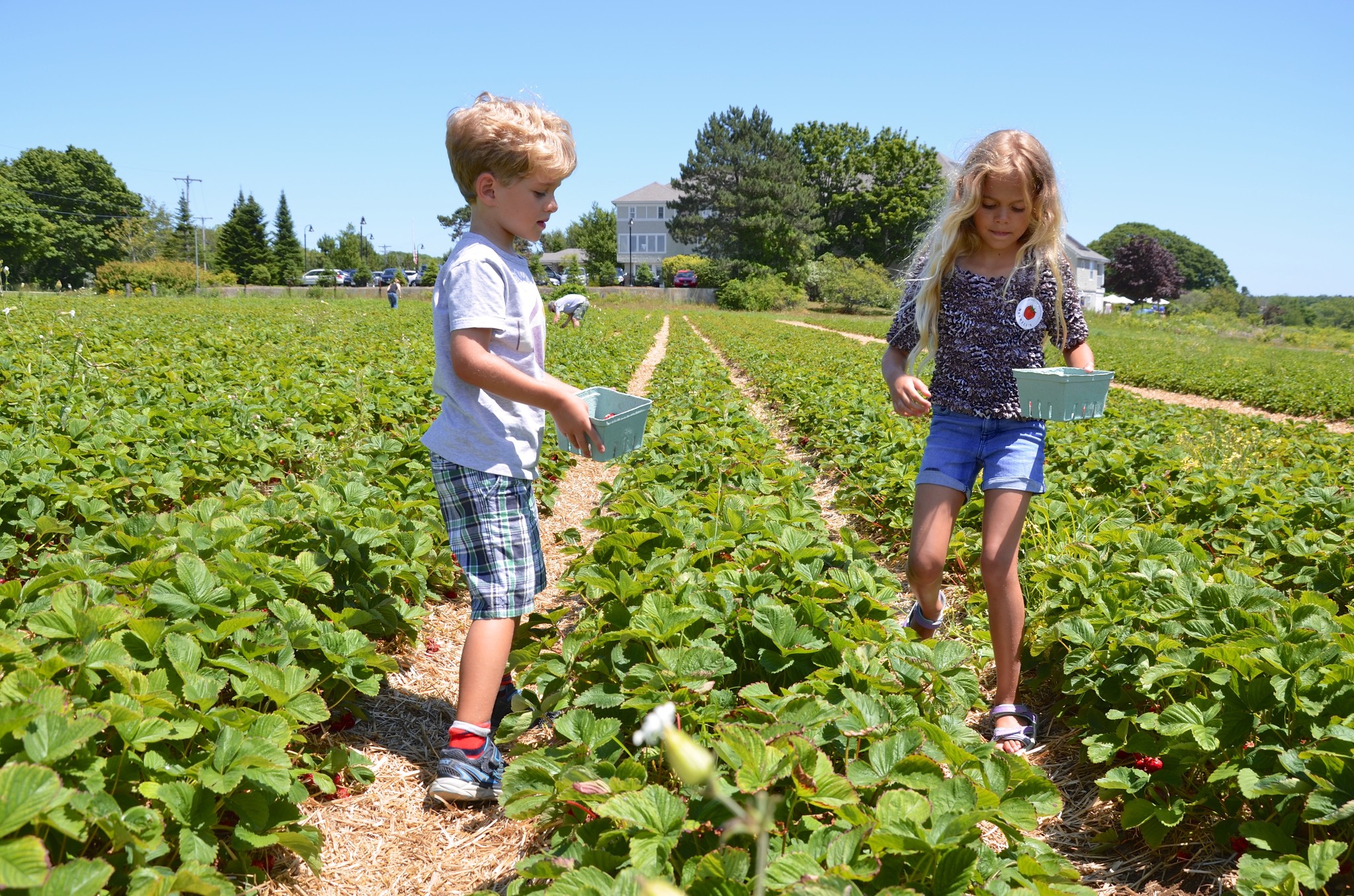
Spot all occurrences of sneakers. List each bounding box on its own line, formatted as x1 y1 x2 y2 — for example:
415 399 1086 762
497 689 560 728
428 733 510 803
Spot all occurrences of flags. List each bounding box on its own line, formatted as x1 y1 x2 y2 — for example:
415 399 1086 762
412 240 418 265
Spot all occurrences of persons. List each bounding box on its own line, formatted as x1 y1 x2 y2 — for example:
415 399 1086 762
880 131 1096 759
417 91 607 799
548 294 590 329
387 278 402 310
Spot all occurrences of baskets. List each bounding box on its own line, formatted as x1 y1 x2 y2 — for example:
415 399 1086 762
1013 366 1114 421
554 386 653 462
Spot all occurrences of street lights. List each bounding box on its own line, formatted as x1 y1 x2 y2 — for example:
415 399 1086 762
417 243 425 271
627 216 634 287
305 224 314 273
365 232 374 263
359 216 367 261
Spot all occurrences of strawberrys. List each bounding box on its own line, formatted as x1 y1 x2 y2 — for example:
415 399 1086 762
0 413 1354 871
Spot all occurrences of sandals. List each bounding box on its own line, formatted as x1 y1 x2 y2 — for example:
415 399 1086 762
903 590 947 630
988 701 1039 755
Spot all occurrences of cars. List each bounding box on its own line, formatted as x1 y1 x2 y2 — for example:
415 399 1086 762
600 267 626 286
299 264 429 287
535 267 590 286
673 269 698 288
632 270 660 287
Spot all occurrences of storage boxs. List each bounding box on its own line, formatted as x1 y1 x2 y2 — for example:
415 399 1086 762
555 385 652 462
1011 365 1116 423
